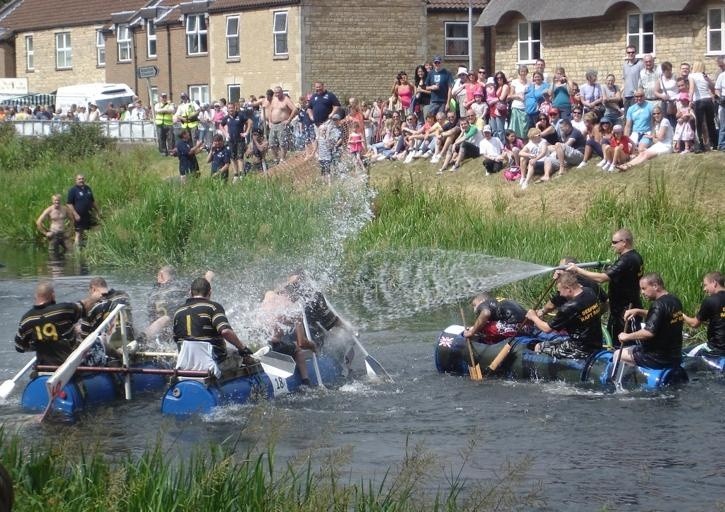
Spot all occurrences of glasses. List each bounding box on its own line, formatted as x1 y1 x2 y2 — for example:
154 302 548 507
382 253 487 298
612 239 623 244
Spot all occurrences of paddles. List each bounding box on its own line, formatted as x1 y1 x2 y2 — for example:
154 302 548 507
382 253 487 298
460 304 484 381
231 347 296 379
1 354 37 399
334 307 393 387
612 302 633 377
488 280 555 370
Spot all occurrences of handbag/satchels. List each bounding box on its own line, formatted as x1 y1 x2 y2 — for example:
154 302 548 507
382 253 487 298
504 166 520 180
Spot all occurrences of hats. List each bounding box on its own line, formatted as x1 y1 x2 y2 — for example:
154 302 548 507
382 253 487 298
473 88 483 95
678 93 690 100
483 125 492 132
456 67 469 76
485 77 495 84
433 56 441 63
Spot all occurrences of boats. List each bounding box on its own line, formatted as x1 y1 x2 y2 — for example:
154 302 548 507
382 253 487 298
433 316 725 398
148 342 354 416
17 342 180 422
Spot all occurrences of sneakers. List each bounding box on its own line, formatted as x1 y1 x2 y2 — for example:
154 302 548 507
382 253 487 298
577 161 588 168
596 159 626 172
391 150 460 171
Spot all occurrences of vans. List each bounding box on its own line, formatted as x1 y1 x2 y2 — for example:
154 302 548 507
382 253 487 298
55 82 141 118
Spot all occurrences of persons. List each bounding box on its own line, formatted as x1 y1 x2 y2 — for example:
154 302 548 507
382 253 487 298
462 227 725 370
2 45 725 188
14 275 136 379
36 194 75 251
146 265 353 389
66 175 102 241
